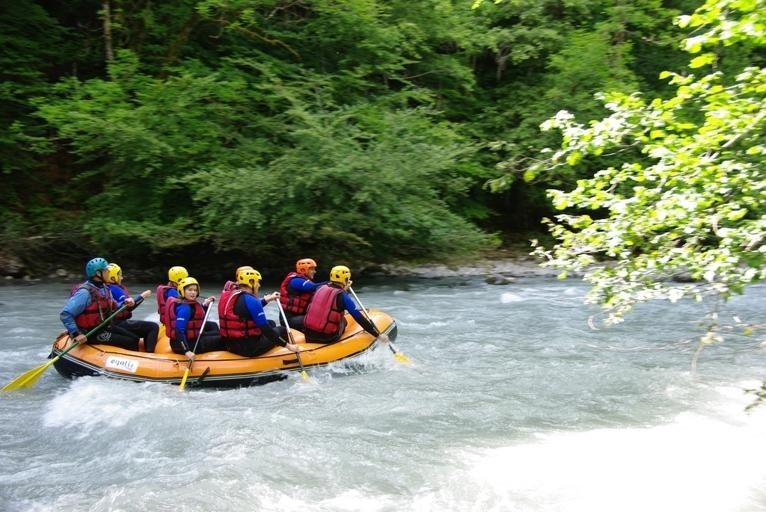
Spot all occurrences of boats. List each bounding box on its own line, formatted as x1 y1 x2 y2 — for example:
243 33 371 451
48 308 400 388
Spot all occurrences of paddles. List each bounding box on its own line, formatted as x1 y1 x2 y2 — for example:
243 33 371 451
275 296 311 383
348 285 410 363
1 303 128 392
178 299 214 393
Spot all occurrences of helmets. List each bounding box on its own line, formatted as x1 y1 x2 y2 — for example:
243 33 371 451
178 277 199 298
235 266 253 280
237 269 263 288
329 265 351 286
167 266 188 284
106 262 121 283
85 257 110 277
296 258 317 276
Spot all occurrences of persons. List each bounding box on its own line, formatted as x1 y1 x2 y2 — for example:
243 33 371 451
217 270 300 358
277 257 330 329
103 264 158 352
305 265 389 343
218 265 272 330
59 258 139 353
165 277 222 358
156 266 218 346
